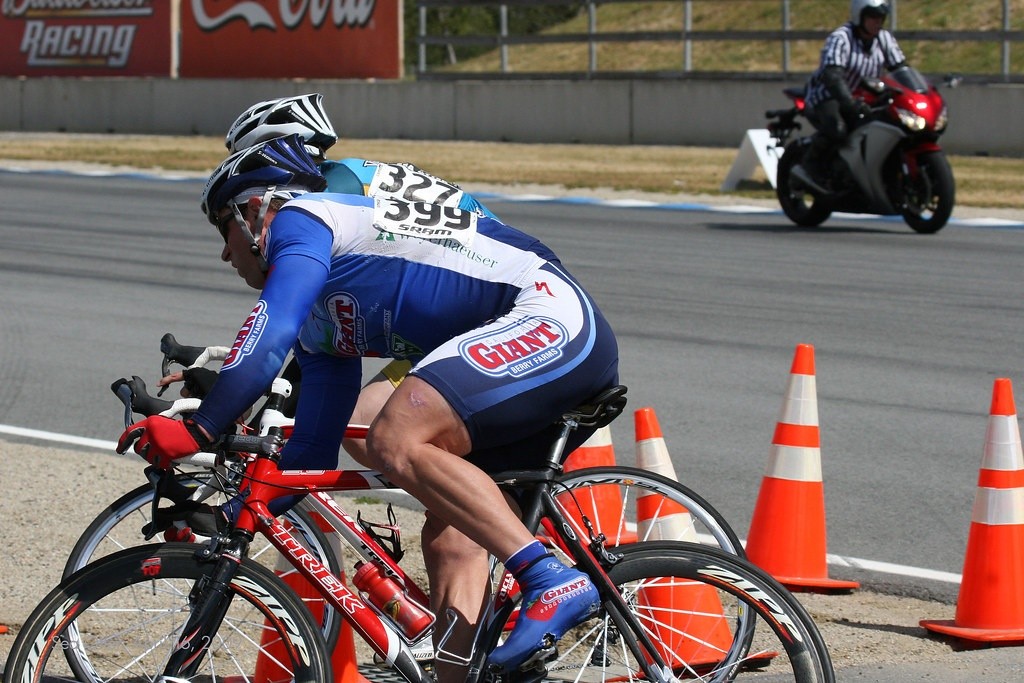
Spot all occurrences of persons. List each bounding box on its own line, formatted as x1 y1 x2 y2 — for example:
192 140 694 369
791 0 908 194
116 92 620 683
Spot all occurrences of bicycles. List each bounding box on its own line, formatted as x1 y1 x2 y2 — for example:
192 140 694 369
0 333 838 683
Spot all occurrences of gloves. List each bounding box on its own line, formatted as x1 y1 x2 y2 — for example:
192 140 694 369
117 415 210 472
142 500 217 542
844 101 872 127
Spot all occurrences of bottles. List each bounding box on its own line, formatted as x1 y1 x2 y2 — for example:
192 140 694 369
351 558 434 643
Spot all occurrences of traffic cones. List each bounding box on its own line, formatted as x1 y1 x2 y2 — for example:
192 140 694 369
919 377 1024 645
223 490 373 683
534 422 640 546
600 407 779 683
744 340 862 590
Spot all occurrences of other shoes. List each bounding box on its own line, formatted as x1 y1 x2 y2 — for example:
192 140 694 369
487 540 600 673
802 146 825 184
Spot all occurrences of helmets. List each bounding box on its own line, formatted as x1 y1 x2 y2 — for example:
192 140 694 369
851 0 890 26
225 93 339 158
200 133 328 226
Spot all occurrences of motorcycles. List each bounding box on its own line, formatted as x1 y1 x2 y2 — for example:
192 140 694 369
764 74 967 233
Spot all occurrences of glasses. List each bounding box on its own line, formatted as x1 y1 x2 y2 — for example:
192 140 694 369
216 204 248 243
